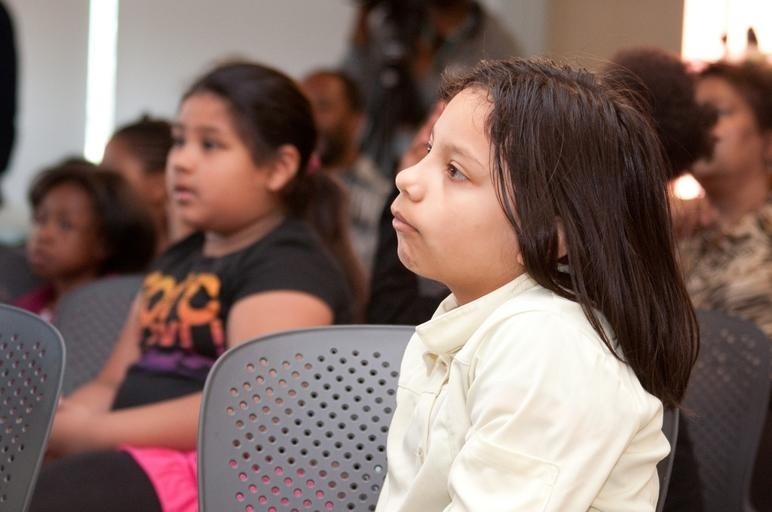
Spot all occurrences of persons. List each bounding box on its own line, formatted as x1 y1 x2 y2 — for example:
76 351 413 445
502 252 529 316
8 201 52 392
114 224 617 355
647 56 770 511
366 94 452 324
370 56 700 510
599 43 719 187
27 64 352 509
343 0 484 99
94 120 171 258
10 157 156 321
295 64 394 326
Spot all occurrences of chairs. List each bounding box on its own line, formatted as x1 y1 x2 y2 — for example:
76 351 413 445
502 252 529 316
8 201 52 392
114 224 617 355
665 306 768 512
0 301 68 512
58 277 146 403
198 325 426 512
